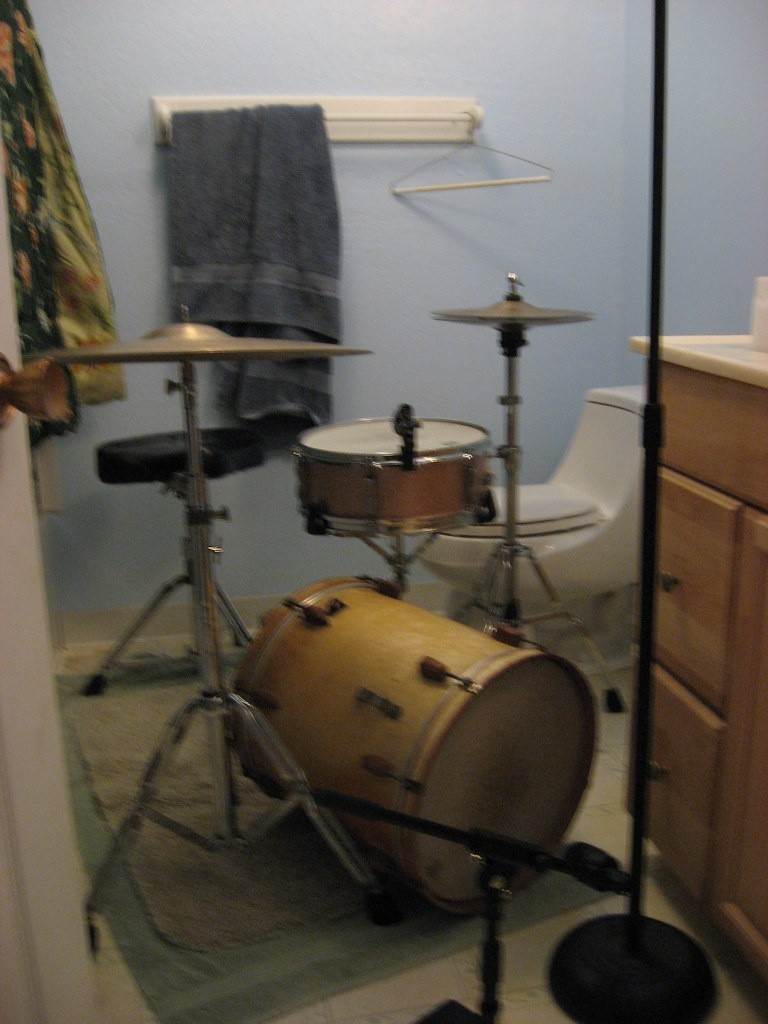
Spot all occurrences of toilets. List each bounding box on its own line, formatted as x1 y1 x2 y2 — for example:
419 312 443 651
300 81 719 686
420 386 647 677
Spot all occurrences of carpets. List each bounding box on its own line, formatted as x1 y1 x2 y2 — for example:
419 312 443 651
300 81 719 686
53 620 616 1024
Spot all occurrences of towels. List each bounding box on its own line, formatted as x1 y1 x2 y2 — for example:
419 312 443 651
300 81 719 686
158 104 344 445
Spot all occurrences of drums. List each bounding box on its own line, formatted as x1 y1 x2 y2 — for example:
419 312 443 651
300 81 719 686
226 575 601 917
294 415 491 536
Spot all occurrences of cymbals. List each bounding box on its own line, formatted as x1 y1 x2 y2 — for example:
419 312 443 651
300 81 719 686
22 322 374 363
428 301 594 325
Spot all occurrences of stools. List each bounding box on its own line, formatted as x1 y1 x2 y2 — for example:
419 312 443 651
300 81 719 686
83 428 275 696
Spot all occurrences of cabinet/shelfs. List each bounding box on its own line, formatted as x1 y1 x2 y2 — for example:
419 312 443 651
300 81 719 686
624 360 768 982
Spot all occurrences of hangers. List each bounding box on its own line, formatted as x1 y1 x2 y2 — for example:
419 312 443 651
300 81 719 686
389 110 555 196
